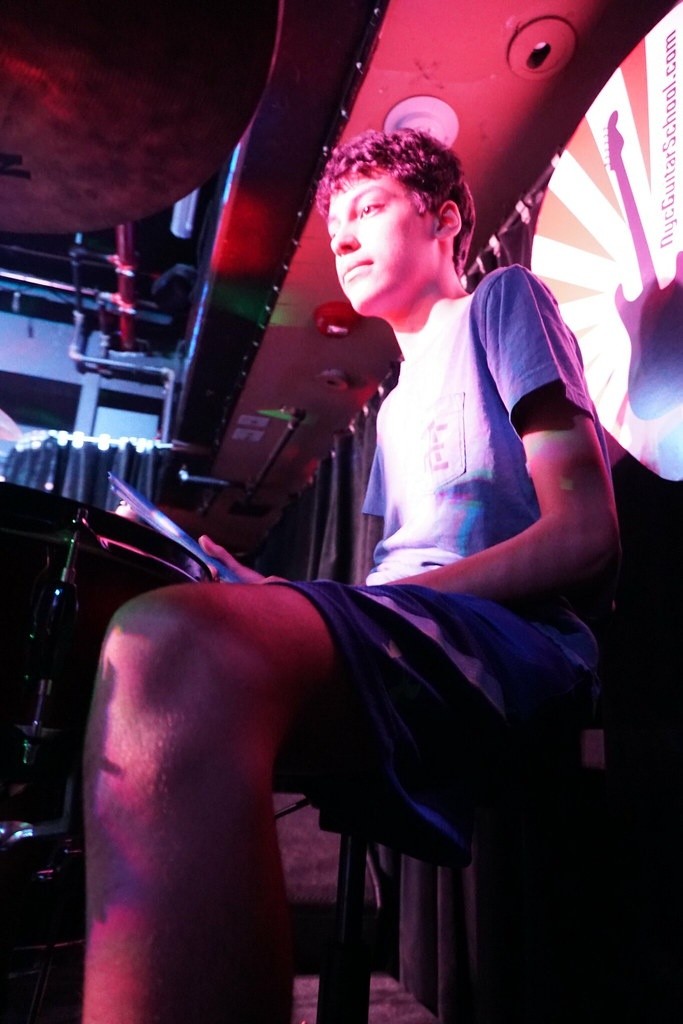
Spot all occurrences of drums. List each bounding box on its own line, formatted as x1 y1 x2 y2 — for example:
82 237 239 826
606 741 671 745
0 480 224 959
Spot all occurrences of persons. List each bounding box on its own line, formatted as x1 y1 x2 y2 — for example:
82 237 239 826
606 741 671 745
84 129 622 1024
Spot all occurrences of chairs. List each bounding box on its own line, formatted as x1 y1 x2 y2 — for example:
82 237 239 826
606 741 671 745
275 449 682 1024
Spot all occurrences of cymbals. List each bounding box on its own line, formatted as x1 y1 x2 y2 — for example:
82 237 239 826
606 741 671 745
0 0 283 238
